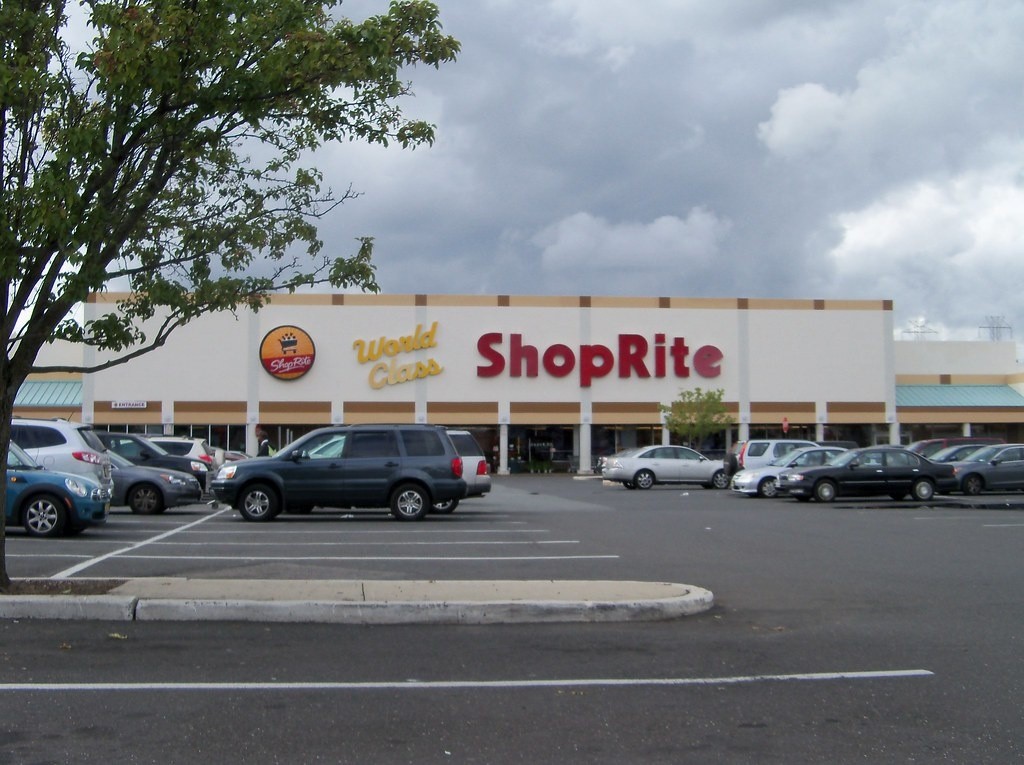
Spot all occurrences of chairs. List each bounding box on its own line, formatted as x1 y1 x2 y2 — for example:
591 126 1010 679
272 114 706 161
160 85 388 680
859 457 872 468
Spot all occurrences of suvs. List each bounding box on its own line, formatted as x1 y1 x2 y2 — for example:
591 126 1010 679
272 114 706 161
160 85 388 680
207 422 466 522
724 438 836 475
307 430 491 513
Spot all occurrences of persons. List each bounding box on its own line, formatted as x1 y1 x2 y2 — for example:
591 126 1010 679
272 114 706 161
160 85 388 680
255 423 277 459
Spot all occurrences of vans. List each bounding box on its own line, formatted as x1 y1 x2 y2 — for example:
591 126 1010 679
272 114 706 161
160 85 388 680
904 438 1007 459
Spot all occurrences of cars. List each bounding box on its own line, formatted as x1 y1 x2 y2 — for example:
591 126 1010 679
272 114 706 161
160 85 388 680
927 445 986 463
951 444 1024 496
3 415 252 539
602 444 731 490
731 446 848 499
774 446 958 503
